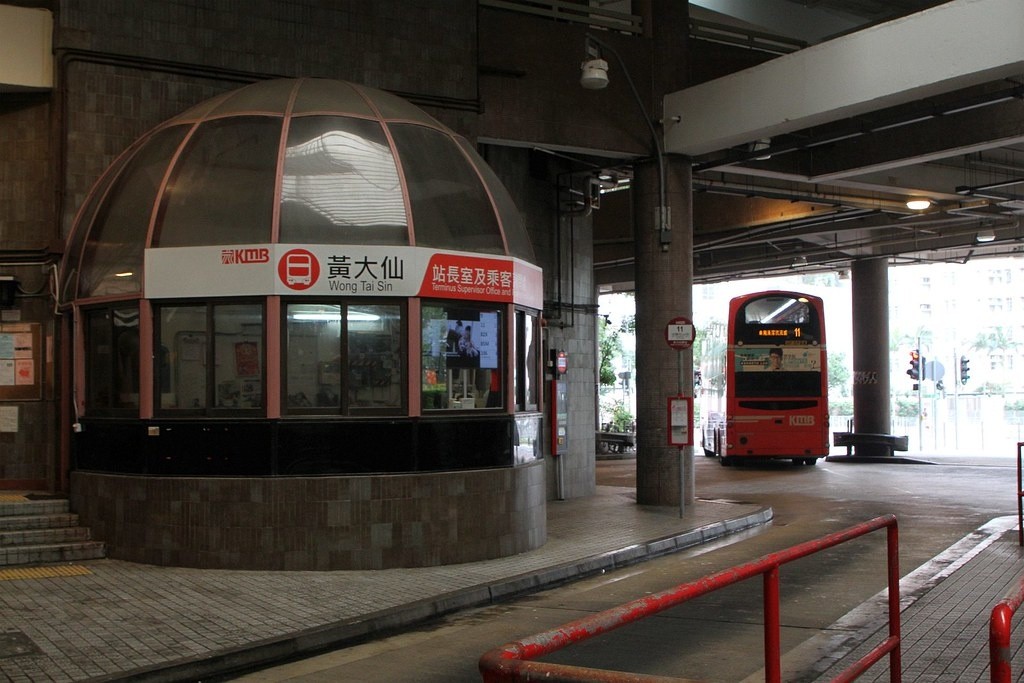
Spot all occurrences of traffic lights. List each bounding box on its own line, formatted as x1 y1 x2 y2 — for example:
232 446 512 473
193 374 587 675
907 350 926 381
960 356 970 385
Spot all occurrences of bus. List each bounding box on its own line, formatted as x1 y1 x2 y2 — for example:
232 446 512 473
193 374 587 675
698 290 830 466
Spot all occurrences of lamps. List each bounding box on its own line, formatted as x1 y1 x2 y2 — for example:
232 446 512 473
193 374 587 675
976 224 995 242
580 55 610 89
905 196 931 210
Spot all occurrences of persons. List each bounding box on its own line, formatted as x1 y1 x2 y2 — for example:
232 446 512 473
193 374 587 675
454 320 474 351
767 348 784 370
